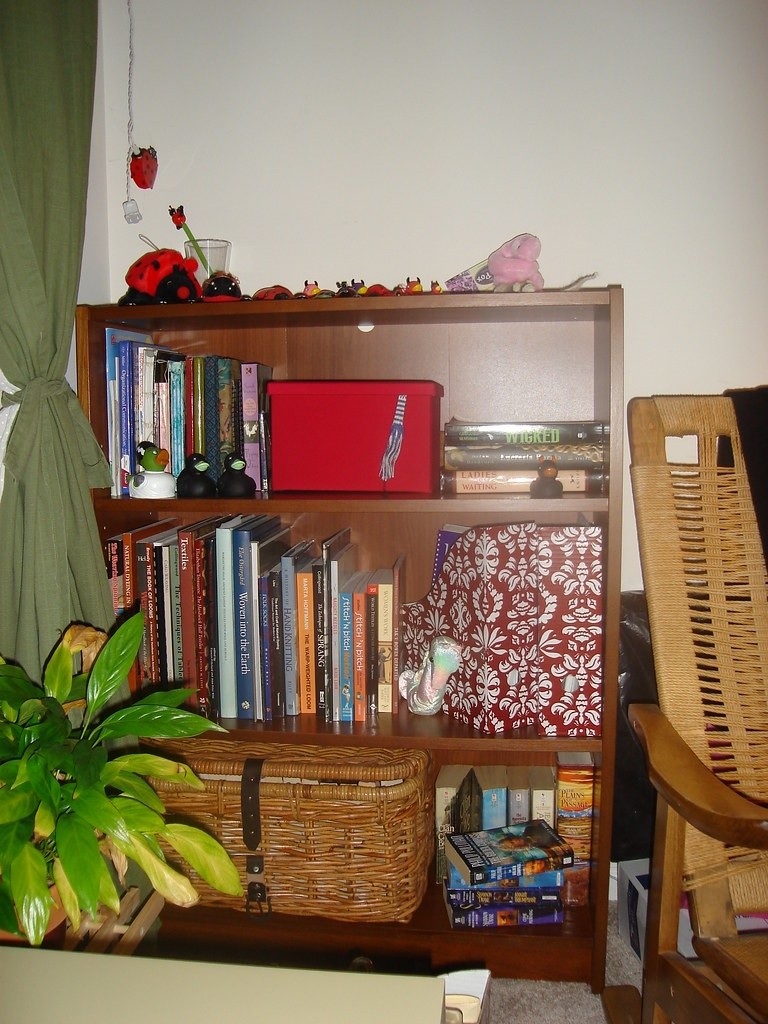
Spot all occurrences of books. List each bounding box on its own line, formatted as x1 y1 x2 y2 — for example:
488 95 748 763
434 752 594 929
103 516 407 725
443 416 601 495
106 324 272 499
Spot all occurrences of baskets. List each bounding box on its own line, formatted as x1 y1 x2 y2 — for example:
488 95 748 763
132 732 434 925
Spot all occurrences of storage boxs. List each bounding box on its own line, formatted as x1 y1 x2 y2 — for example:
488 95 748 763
147 739 431 922
615 857 767 965
267 381 443 495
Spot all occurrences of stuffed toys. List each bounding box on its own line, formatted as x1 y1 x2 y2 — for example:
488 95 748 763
399 636 461 716
488 233 545 293
118 249 306 304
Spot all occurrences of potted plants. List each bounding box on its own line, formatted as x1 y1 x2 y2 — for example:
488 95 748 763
0 608 244 949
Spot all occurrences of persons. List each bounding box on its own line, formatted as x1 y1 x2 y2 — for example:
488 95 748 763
525 859 562 873
498 822 566 850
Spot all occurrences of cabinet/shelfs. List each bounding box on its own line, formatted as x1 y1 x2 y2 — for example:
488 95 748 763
71 284 628 995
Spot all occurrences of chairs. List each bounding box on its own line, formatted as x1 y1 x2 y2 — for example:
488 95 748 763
631 394 768 1024
